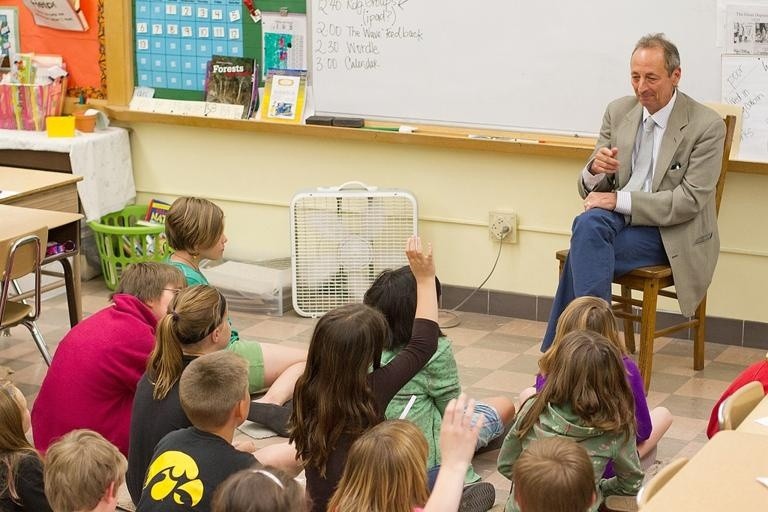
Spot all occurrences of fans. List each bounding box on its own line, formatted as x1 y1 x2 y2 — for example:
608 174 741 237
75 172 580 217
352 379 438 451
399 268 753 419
288 180 422 320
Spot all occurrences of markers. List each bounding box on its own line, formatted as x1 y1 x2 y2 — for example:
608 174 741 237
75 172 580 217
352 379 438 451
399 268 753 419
466 134 546 143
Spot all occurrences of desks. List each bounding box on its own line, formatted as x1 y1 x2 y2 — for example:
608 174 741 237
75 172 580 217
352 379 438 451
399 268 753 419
0 200 87 351
1 124 138 283
1 157 85 327
732 392 766 434
634 426 767 511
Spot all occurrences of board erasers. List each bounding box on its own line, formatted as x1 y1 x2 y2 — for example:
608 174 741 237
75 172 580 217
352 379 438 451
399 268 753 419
305 116 364 128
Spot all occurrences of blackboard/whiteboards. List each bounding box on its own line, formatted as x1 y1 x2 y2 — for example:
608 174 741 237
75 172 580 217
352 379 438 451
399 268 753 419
305 0 727 139
719 52 768 164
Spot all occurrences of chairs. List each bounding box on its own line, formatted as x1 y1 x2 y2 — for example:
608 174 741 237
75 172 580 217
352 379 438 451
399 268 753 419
558 112 739 397
0 225 58 378
634 453 688 508
713 380 764 431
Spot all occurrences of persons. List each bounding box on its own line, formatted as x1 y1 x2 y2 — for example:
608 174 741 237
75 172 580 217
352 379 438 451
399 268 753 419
539 31 721 354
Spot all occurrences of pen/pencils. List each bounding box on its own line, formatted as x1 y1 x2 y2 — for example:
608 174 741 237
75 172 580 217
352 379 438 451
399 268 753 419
364 126 416 133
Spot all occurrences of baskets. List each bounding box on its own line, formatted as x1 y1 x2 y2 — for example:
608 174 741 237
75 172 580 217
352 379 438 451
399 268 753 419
85 204 174 291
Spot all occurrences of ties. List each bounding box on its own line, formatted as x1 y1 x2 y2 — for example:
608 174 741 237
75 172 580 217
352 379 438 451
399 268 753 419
620 115 656 193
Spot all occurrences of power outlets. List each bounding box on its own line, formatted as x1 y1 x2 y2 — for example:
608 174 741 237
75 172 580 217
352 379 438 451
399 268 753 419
486 209 519 245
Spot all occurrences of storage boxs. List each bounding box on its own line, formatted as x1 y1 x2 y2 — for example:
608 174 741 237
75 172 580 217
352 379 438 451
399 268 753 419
0 74 72 133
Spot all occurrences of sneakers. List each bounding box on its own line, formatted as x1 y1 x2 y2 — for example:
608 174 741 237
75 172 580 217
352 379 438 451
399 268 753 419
246 401 295 438
604 493 640 511
457 480 496 511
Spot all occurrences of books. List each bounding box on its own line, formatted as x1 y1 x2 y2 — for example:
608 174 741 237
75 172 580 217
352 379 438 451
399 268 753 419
204 54 308 125
